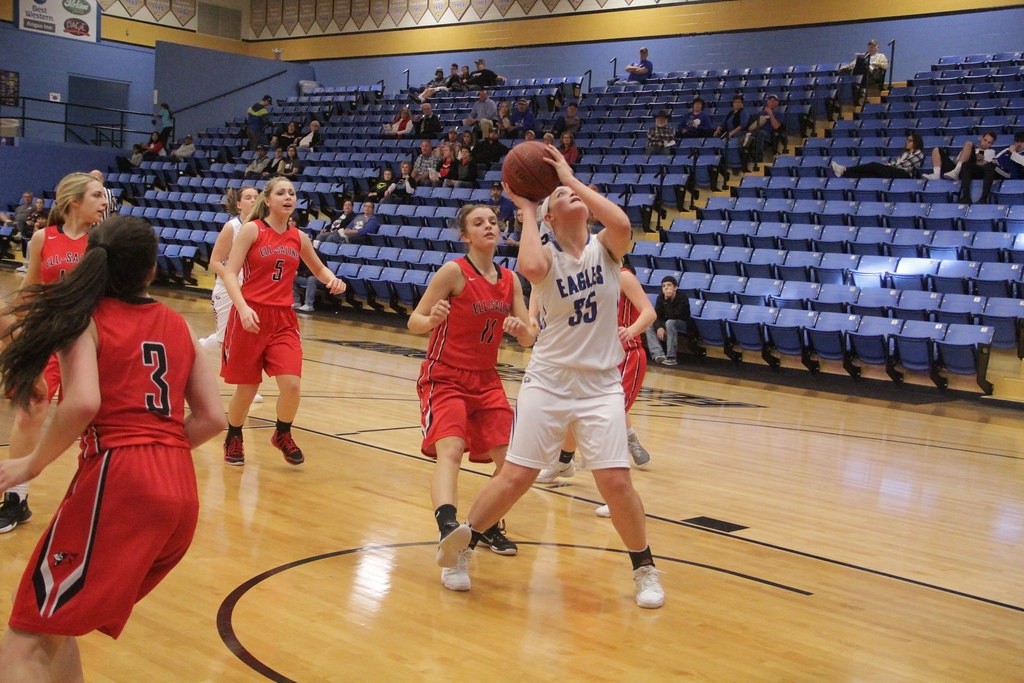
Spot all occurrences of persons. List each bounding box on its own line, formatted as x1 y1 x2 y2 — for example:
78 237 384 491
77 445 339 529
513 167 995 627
614 47 653 85
644 109 676 155
115 131 196 168
245 94 273 148
198 175 347 464
289 197 381 244
231 121 325 180
352 127 510 205
152 102 174 145
713 95 750 138
290 231 329 311
489 179 523 257
0 171 228 683
407 143 666 609
675 97 712 138
922 131 997 180
747 93 783 162
0 191 48 273
507 97 583 166
954 131 1024 204
645 276 701 366
588 184 598 194
839 39 889 87
89 169 118 225
381 58 507 136
831 133 925 179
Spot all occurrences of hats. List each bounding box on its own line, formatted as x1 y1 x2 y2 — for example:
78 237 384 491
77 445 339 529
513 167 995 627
766 94 780 101
475 58 485 65
517 98 527 105
868 39 878 46
449 127 457 133
640 47 648 53
265 95 273 105
256 144 265 152
654 110 670 119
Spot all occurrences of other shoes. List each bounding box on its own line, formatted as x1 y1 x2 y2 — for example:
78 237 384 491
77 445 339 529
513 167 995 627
298 305 314 311
253 394 263 403
661 359 677 365
199 338 205 344
16 266 28 273
831 161 997 207
655 355 666 364
291 303 301 310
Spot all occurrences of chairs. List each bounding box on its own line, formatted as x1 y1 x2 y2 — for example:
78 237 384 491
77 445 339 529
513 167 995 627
0 53 1023 401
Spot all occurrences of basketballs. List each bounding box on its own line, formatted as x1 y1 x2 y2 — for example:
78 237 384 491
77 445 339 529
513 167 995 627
503 141 560 201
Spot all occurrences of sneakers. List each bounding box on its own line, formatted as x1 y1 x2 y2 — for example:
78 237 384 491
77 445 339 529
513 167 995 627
633 565 667 609
0 492 32 533
596 503 612 518
271 429 304 465
626 432 651 465
436 520 472 567
476 518 518 555
441 547 473 590
223 430 246 466
535 459 575 483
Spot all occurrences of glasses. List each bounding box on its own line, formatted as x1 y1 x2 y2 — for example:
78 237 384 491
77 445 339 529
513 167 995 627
905 138 914 143
